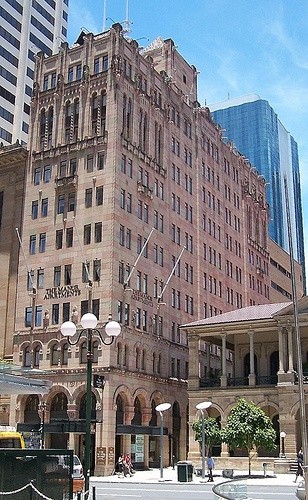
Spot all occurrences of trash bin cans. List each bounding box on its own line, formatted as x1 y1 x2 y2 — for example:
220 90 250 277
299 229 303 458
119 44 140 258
177 460 194 482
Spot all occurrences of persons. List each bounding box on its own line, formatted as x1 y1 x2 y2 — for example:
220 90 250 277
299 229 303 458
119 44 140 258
115 454 135 478
206 455 215 483
293 447 304 483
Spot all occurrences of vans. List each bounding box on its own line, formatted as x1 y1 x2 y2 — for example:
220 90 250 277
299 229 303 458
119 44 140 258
0 431 25 460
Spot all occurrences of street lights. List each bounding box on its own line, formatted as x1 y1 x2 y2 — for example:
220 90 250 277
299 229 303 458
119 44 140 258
196 402 212 484
60 313 121 479
154 402 171 482
39 401 46 450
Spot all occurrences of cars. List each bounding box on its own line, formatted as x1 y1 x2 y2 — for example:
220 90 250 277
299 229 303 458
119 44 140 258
26 455 84 481
